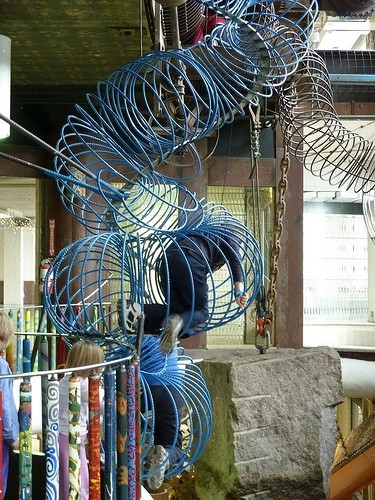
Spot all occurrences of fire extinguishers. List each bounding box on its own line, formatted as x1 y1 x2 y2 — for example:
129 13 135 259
39 256 55 295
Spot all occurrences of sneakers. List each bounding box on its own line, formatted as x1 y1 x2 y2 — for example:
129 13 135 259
145 443 169 491
117 299 142 334
162 315 186 354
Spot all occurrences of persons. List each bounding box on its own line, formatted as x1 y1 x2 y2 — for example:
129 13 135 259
116 219 248 356
62 340 106 500
0 310 21 500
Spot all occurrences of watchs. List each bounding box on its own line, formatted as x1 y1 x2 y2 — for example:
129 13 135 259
234 290 243 298
